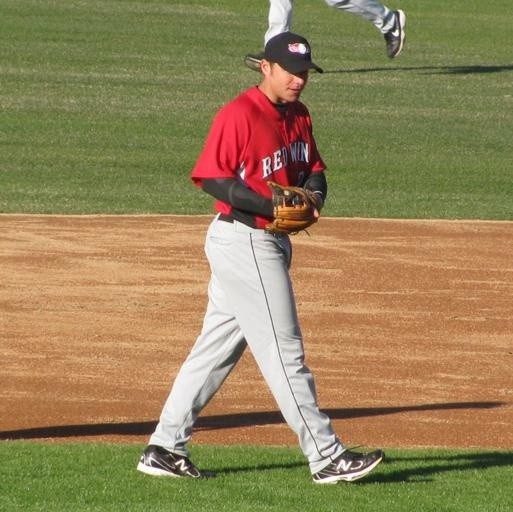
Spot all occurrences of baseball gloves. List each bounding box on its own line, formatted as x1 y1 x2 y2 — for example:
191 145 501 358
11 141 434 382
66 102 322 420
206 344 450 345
264 180 323 237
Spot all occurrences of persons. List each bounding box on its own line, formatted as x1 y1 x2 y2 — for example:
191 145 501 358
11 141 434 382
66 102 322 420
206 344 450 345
135 30 386 486
242 1 407 74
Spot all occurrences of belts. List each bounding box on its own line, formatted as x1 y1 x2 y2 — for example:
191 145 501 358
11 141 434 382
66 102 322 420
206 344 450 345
218 213 234 224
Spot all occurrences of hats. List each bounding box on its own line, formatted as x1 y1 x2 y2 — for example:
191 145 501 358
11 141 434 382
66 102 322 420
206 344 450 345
265 31 323 74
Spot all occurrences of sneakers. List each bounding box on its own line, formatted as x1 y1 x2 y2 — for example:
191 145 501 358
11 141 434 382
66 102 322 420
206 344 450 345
384 10 406 58
136 444 215 479
313 450 384 485
244 52 265 73
